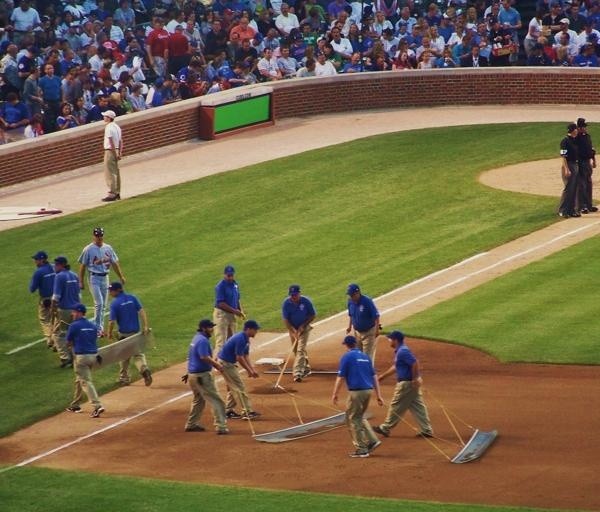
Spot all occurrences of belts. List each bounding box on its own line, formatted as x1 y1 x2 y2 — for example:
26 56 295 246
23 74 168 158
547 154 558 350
88 271 109 276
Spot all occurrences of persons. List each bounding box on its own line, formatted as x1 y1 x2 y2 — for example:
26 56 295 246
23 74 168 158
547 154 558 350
99 109 124 201
78 226 125 338
576 117 599 214
281 284 317 382
47 256 81 368
331 335 385 459
214 319 262 421
28 250 57 351
556 124 583 219
372 329 435 438
211 264 247 374
103 281 153 387
345 283 381 368
180 319 230 435
1 2 599 146
64 302 105 418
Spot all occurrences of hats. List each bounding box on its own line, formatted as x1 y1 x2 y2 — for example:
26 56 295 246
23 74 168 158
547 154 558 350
165 73 178 81
345 284 361 296
341 335 357 346
255 32 265 42
54 256 68 264
31 251 49 260
293 32 303 40
199 319 217 329
102 41 113 50
559 17 571 26
576 118 587 128
399 21 409 27
568 124 577 133
154 77 165 84
488 17 498 27
244 319 262 330
224 265 235 276
386 330 405 341
94 227 104 236
69 303 87 313
107 281 123 290
288 285 301 296
100 109 117 120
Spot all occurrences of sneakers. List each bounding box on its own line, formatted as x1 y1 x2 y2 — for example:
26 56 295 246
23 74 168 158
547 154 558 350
347 449 371 458
216 427 230 435
63 403 84 413
372 424 391 438
301 369 311 378
578 208 590 214
46 339 58 353
184 424 205 432
241 411 262 420
557 211 569 218
142 368 153 387
293 375 302 383
115 193 121 200
95 329 108 338
118 380 131 387
89 405 105 419
225 411 242 420
586 206 598 212
59 359 72 369
415 430 434 439
366 436 382 453
567 210 581 217
101 195 117 202
